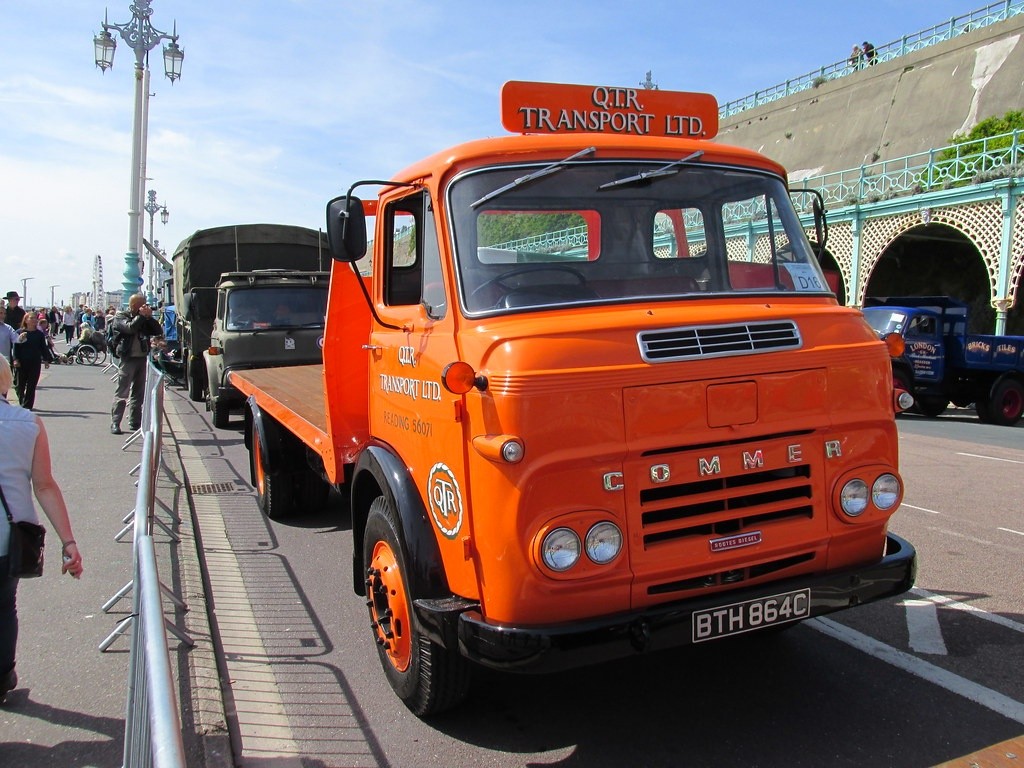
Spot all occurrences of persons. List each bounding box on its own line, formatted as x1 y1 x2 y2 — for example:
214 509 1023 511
4 291 25 331
0 299 27 398
31 305 115 363
13 312 50 409
151 337 184 383
111 294 163 435
862 42 878 66
850 45 863 71
0 355 82 703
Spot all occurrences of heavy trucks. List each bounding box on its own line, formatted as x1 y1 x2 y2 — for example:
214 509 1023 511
171 223 331 429
862 295 1023 425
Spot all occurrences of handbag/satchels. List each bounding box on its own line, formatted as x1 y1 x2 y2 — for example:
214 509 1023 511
7 520 47 579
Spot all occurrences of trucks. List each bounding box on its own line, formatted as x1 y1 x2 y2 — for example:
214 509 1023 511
225 80 924 728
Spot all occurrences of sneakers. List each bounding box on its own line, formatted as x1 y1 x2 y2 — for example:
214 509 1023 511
129 424 141 430
111 423 121 434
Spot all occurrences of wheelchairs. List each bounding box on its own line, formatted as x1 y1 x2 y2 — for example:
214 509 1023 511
43 336 61 365
68 331 108 366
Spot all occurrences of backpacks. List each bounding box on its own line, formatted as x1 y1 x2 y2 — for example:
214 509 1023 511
107 312 131 359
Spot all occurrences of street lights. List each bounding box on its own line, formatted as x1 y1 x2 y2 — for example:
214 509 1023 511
142 188 171 312
50 285 61 308
145 240 167 308
92 0 185 311
21 277 35 310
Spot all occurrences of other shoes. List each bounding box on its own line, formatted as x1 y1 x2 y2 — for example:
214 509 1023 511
67 343 71 346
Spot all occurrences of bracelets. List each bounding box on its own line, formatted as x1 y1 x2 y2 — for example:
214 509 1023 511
63 541 76 548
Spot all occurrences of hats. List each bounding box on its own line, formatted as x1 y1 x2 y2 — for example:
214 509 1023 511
2 291 24 299
